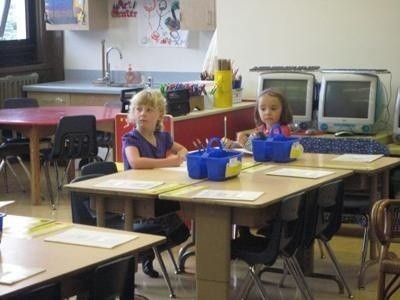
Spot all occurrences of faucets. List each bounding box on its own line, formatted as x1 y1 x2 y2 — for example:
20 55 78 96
102 46 122 85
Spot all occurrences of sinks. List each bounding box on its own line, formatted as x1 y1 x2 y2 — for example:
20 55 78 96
112 83 139 88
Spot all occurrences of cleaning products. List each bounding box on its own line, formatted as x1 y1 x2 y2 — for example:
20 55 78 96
125 64 135 83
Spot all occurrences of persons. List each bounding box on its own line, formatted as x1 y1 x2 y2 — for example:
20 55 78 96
122 89 191 277
220 86 294 149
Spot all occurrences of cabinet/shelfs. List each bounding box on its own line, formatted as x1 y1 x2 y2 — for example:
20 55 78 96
44 0 109 32
69 94 120 107
26 91 71 106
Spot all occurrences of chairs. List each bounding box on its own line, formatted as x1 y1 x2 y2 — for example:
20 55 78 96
299 178 354 298
69 174 190 299
83 162 121 180
0 99 48 193
228 192 315 300
372 194 400 300
39 115 102 210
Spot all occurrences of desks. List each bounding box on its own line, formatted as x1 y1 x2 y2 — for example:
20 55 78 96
160 163 354 300
260 152 400 252
0 104 120 207
64 154 260 267
0 212 168 300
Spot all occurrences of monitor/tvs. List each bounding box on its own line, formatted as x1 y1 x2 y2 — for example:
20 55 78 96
318 74 386 135
257 73 315 129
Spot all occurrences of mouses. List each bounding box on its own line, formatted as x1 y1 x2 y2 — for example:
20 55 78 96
335 130 352 136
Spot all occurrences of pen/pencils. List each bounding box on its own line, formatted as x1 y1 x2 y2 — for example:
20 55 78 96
200 70 214 81
233 67 239 79
159 82 217 97
217 59 231 70
224 116 227 139
190 138 211 154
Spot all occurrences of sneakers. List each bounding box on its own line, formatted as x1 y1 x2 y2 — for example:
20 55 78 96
142 260 159 278
